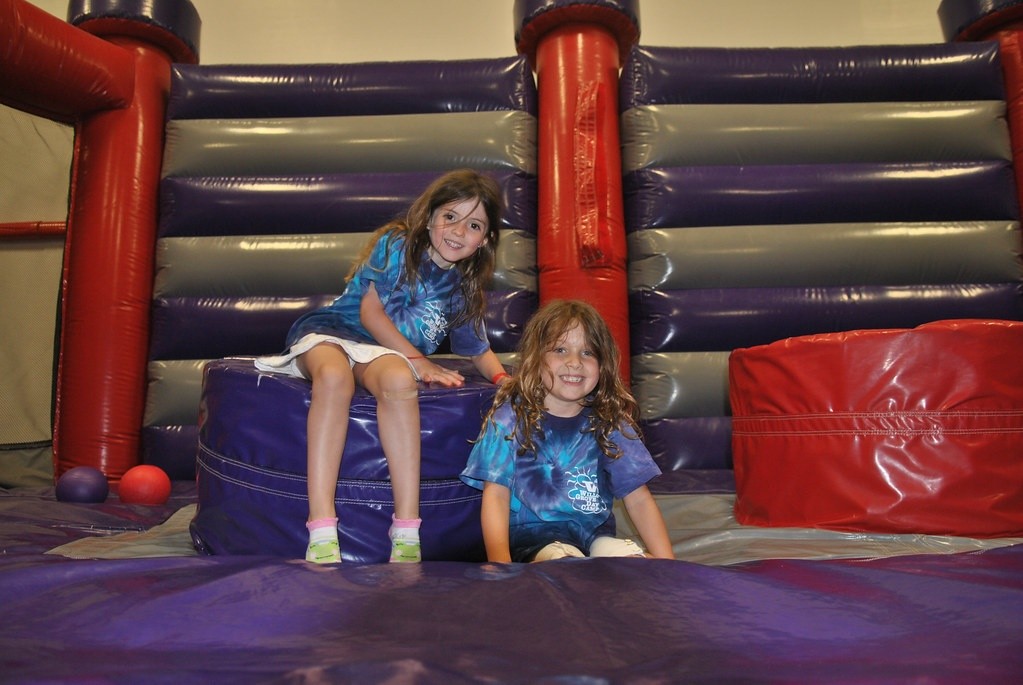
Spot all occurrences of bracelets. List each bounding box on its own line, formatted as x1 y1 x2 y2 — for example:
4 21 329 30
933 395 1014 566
493 372 512 384
407 356 426 359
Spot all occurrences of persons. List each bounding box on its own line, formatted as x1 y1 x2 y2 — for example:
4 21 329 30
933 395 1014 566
457 299 676 562
253 169 516 568
